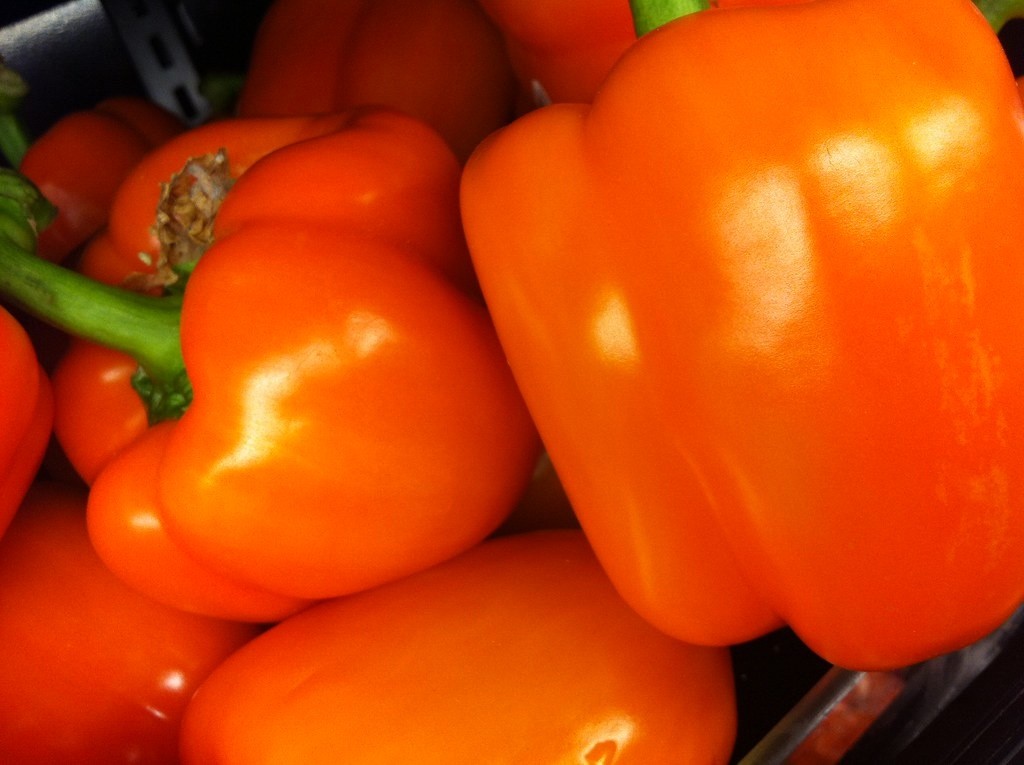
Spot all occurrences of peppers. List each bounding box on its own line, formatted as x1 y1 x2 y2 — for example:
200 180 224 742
0 0 1024 765
